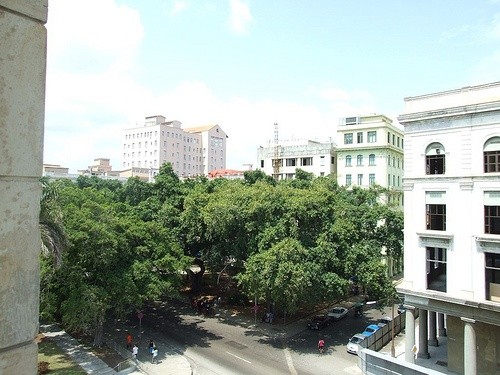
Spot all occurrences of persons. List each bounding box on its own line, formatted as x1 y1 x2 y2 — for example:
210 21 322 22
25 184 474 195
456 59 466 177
149 340 153 355
151 347 159 364
132 344 138 358
318 337 324 354
126 332 132 350
259 311 273 324
190 295 222 317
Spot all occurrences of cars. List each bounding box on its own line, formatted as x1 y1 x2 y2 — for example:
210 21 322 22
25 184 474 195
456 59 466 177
306 314 331 331
360 324 384 338
327 306 349 322
376 316 393 328
346 333 368 356
396 304 407 314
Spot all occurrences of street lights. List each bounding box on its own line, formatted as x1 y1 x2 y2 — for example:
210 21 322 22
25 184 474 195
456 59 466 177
214 259 234 303
